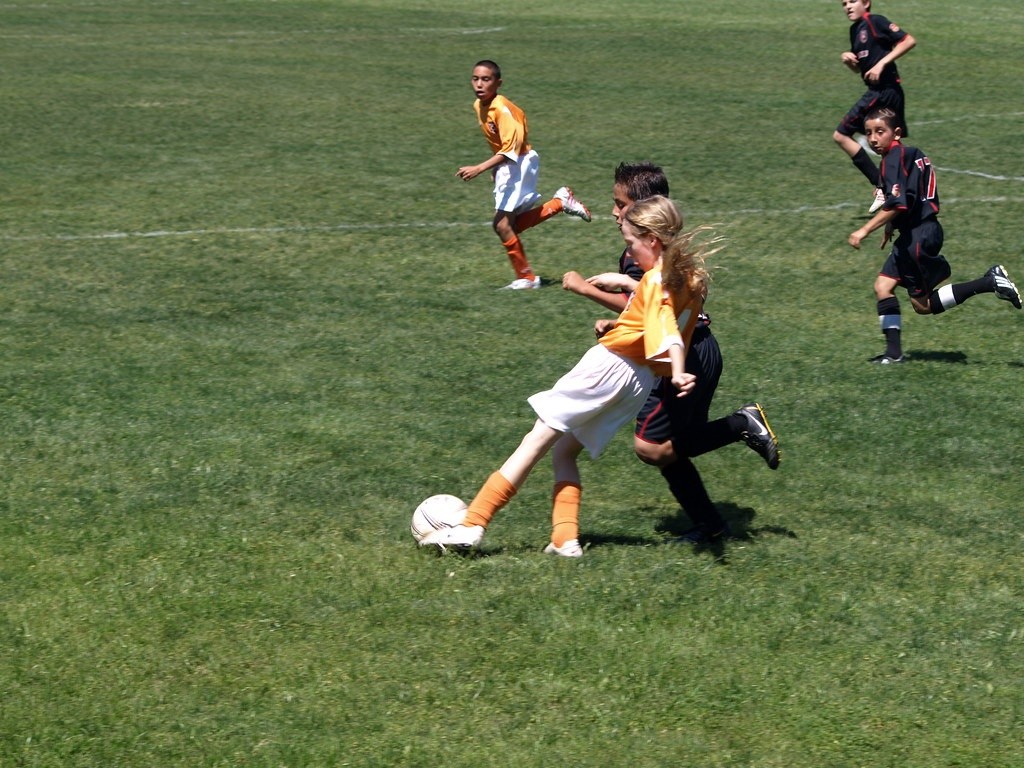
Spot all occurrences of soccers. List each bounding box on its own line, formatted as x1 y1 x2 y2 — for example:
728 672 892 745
408 494 472 547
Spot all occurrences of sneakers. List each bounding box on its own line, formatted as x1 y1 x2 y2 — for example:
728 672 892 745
679 521 733 545
417 525 485 557
544 538 583 557
869 189 886 213
732 403 781 470
868 355 905 364
506 276 541 289
553 187 591 223
984 265 1022 309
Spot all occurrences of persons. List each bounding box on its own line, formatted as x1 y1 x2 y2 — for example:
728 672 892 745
833 0 917 214
848 107 1022 366
454 60 592 290
415 195 729 556
584 163 782 547
